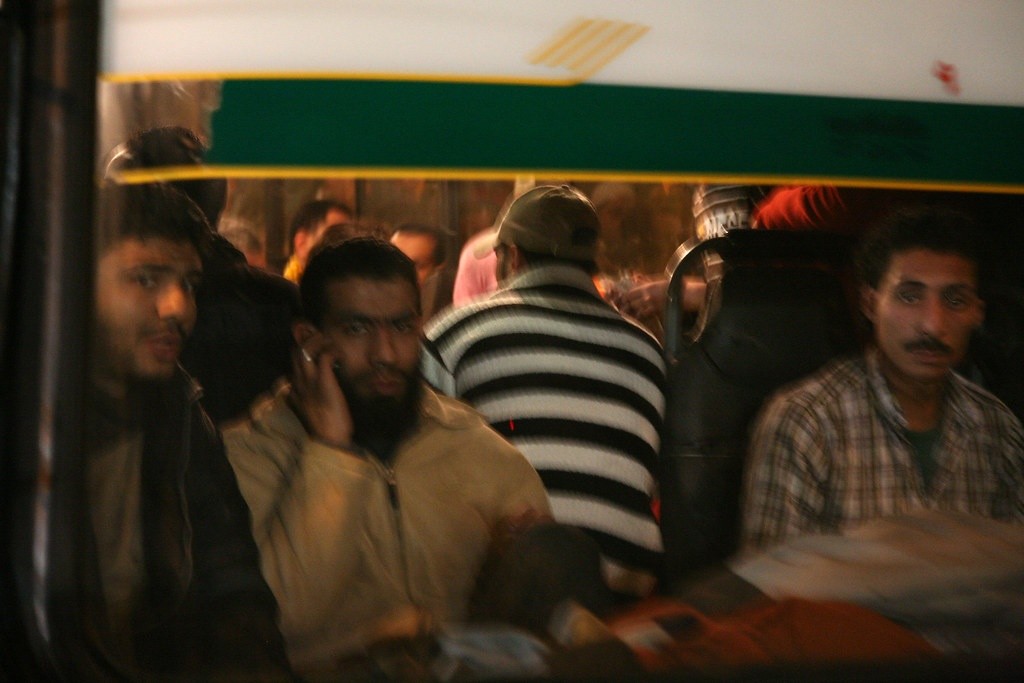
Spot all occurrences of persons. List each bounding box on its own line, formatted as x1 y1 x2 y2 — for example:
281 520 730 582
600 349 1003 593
79 125 1024 683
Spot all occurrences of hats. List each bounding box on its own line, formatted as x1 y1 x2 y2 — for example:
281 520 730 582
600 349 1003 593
475 184 600 260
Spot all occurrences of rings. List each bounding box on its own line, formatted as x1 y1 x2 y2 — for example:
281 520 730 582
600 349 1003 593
302 348 311 362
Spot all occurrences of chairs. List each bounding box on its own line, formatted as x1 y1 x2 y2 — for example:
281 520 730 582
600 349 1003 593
665 229 859 575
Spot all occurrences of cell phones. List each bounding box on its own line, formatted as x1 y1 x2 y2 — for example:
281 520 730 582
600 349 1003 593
312 354 347 393
